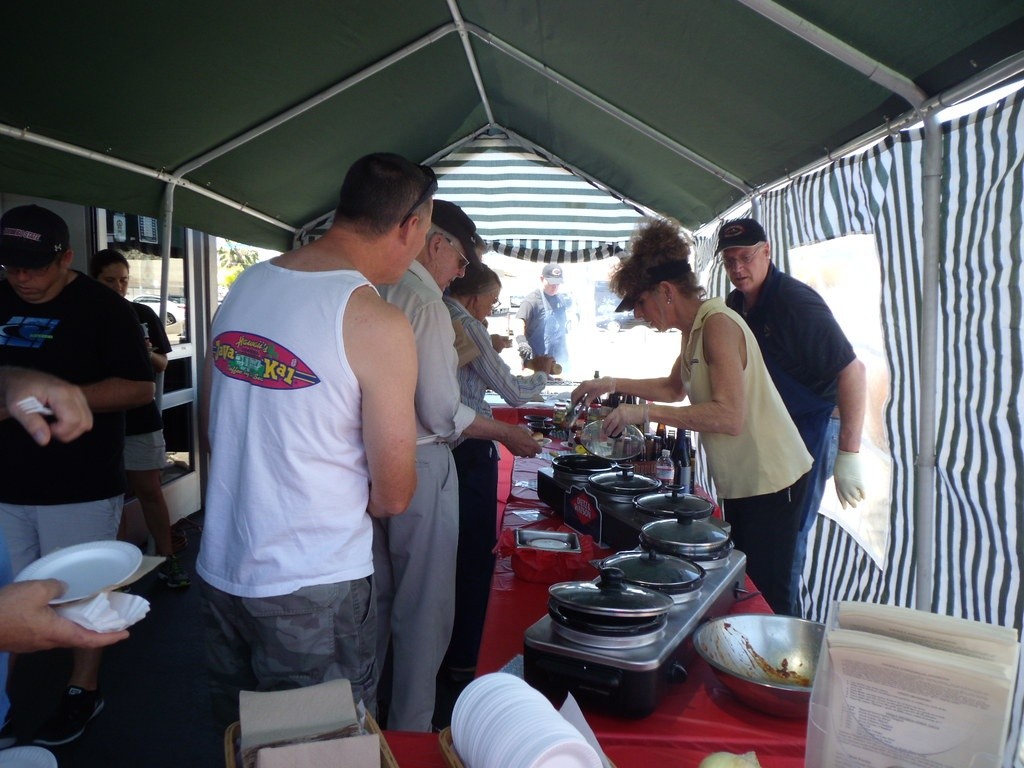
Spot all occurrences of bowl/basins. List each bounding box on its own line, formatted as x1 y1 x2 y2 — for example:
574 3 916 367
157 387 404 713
691 611 826 720
524 415 548 421
527 422 556 434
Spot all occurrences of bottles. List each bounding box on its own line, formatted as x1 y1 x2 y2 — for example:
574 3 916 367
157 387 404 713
552 371 696 493
523 359 562 375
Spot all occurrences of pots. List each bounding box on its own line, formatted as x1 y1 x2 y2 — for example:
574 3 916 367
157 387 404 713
587 463 663 497
599 547 706 595
546 567 675 637
638 514 734 561
551 453 618 475
632 484 714 520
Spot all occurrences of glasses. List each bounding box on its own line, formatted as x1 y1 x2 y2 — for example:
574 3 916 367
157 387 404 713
399 165 438 228
491 293 501 310
434 231 471 270
0 248 67 277
720 242 766 269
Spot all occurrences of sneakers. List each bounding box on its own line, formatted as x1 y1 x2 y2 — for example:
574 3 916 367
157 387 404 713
158 553 192 588
115 586 131 593
33 685 105 745
0 716 18 749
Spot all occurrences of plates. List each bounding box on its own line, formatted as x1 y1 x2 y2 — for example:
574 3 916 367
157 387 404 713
53 591 151 634
13 539 143 605
450 672 604 768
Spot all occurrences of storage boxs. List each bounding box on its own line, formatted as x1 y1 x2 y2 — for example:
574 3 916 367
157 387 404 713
804 600 1024 768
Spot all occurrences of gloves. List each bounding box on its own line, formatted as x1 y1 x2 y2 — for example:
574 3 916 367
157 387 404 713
516 335 533 360
833 449 866 510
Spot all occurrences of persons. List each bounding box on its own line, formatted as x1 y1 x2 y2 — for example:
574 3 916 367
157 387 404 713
196 154 438 725
713 219 867 618
371 199 555 733
571 219 815 618
0 366 130 731
513 264 573 373
0 205 191 748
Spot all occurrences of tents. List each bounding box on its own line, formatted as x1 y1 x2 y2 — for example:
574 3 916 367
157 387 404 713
0 1 1024 768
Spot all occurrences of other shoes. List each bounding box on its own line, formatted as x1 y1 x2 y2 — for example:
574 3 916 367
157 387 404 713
445 665 477 682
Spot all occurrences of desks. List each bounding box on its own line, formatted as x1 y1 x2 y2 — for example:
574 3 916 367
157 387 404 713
382 384 808 768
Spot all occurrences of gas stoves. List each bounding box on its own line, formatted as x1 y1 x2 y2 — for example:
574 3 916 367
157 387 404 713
537 466 731 550
521 549 747 722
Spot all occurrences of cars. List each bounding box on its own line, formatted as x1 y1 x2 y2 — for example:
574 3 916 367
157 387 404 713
128 295 186 337
595 309 661 333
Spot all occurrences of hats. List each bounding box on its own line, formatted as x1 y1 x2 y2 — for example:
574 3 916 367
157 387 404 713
0 204 70 269
615 259 691 312
717 219 767 251
543 263 563 284
431 200 484 273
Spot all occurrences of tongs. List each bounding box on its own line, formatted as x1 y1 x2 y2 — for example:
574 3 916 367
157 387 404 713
564 393 585 427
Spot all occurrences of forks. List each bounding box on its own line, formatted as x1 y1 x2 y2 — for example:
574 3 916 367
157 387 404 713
17 395 53 416
